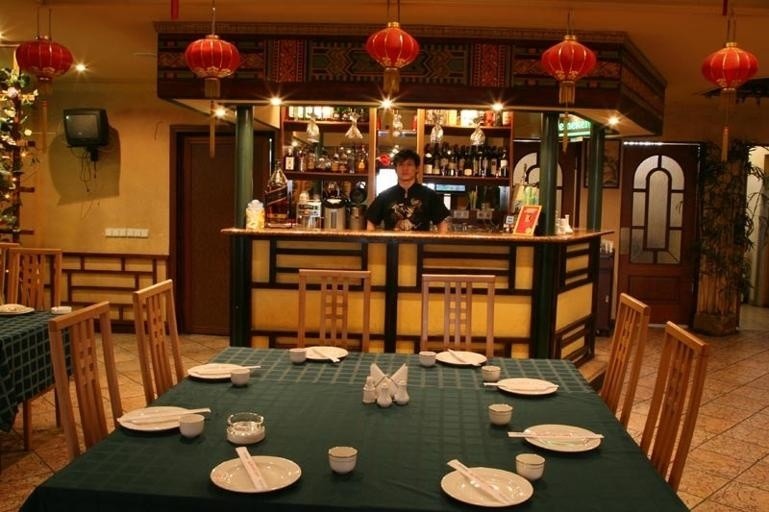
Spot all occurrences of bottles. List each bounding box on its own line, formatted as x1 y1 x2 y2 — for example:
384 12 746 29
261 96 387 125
422 141 509 178
495 112 502 127
377 383 392 408
412 114 417 131
509 207 519 231
284 141 368 174
263 159 291 224
362 376 376 404
476 183 500 211
520 163 527 184
394 380 410 405
360 108 366 122
376 115 381 131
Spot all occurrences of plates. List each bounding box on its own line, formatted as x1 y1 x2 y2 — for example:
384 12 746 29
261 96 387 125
435 351 487 365
523 425 601 453
0 305 35 315
303 346 349 360
187 364 243 379
210 456 303 493
497 378 558 395
439 468 534 507
119 406 190 431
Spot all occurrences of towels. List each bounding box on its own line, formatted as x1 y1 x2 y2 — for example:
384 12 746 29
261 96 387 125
391 365 408 398
370 362 389 397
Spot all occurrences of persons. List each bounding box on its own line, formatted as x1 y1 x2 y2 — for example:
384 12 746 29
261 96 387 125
364 149 451 232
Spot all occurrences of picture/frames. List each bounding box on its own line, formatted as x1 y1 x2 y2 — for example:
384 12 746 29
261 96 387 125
512 204 543 236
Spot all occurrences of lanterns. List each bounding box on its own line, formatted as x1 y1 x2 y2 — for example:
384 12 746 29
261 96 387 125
365 21 420 93
541 34 596 151
184 34 241 157
700 42 760 162
16 34 74 153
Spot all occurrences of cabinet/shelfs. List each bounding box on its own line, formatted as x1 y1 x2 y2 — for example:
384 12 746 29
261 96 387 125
416 108 514 229
279 104 376 208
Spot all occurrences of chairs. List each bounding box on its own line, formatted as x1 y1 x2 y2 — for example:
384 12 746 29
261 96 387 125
420 274 496 357
0 241 20 304
638 320 710 494
7 247 63 311
132 279 185 406
600 291 652 431
47 299 123 463
298 268 372 353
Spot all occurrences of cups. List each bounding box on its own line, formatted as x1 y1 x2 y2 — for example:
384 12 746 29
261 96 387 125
487 403 513 425
419 351 437 367
300 215 322 232
515 453 545 482
327 445 359 473
347 204 366 230
179 413 205 438
288 348 306 364
230 367 251 386
227 413 266 444
555 214 574 235
481 365 501 382
323 198 346 231
